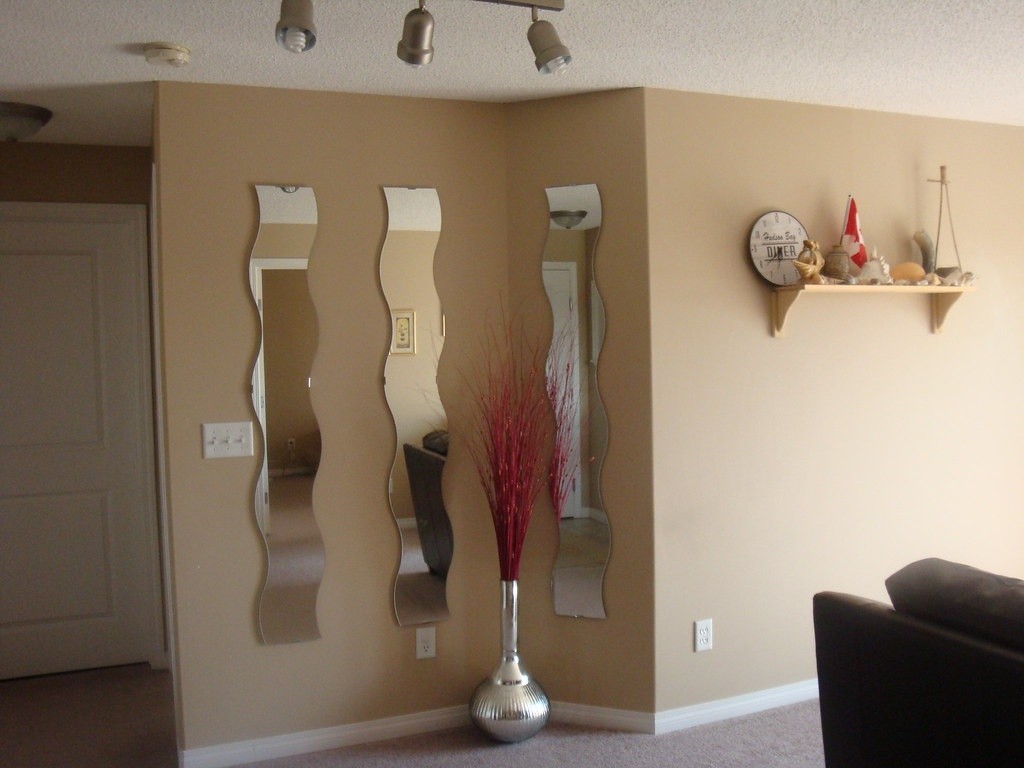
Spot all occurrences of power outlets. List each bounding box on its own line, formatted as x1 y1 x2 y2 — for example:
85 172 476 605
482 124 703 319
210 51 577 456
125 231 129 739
416 628 436 658
694 619 713 652
288 439 296 450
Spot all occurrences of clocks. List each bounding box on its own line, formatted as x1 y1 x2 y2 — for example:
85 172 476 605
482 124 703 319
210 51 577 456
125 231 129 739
747 209 811 287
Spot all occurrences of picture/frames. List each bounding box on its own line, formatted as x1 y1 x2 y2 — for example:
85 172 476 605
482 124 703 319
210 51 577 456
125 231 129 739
389 309 416 355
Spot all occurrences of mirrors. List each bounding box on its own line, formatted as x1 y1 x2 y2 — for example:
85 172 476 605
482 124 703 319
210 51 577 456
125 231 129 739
380 185 455 626
540 182 606 619
247 180 328 641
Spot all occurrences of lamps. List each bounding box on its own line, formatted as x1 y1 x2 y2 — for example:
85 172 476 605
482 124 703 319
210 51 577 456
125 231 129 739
0 102 53 136
550 209 586 230
276 0 573 82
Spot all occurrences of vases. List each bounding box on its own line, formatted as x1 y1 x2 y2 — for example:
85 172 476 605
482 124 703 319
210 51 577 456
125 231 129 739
472 581 551 745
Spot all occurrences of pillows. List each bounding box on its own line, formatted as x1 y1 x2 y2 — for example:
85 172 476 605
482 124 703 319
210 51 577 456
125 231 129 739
422 430 449 456
885 558 1024 647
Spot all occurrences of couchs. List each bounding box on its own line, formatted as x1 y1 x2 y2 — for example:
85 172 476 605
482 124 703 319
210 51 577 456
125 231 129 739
812 557 1024 768
401 429 453 579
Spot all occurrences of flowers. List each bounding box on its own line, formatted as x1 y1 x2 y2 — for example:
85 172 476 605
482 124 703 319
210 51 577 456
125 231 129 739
447 291 563 580
